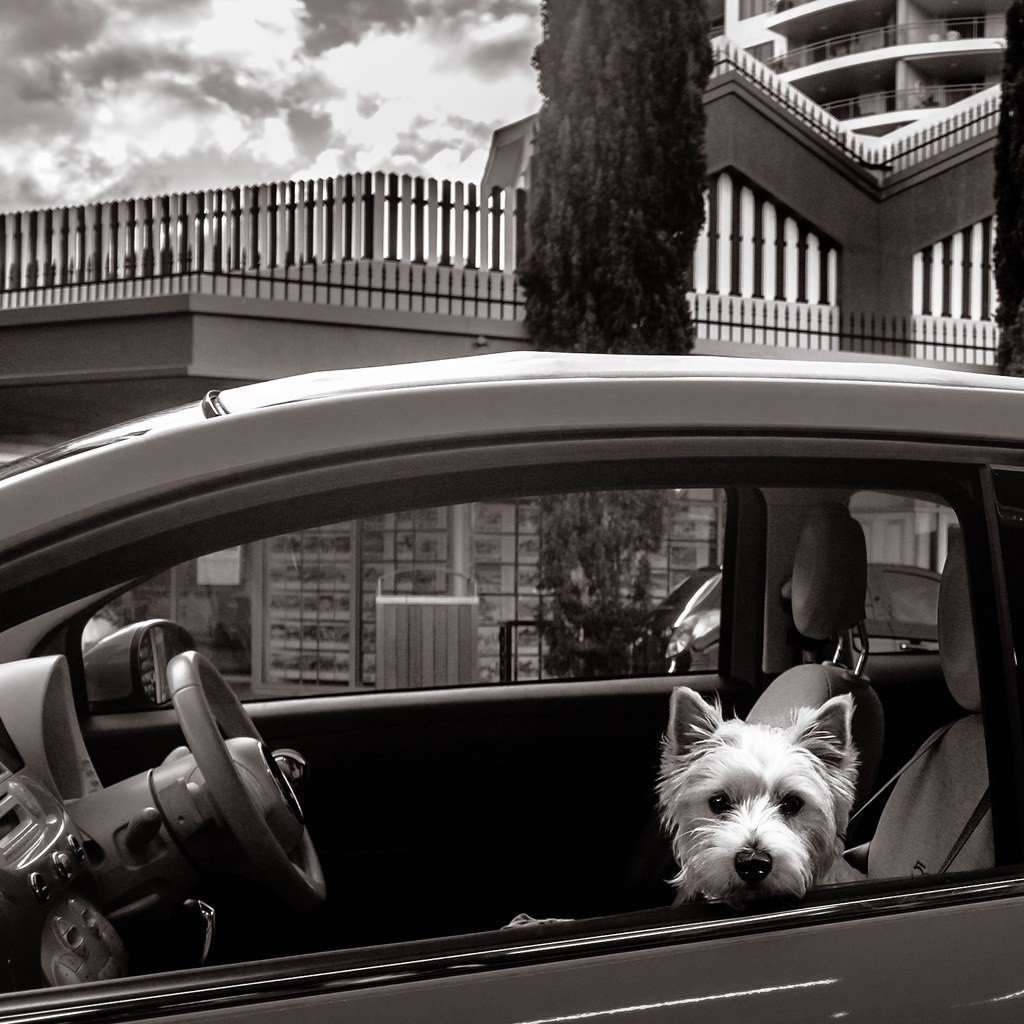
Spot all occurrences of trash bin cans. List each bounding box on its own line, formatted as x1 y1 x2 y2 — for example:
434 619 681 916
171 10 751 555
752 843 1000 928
374 565 480 692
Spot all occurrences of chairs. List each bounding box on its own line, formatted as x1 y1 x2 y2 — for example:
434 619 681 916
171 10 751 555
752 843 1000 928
843 533 995 887
745 505 887 851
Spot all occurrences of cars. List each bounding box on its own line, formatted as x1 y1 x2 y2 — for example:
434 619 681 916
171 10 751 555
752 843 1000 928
1 353 1024 1024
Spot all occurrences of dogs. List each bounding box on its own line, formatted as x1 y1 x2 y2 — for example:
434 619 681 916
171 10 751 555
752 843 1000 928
655 686 864 912
